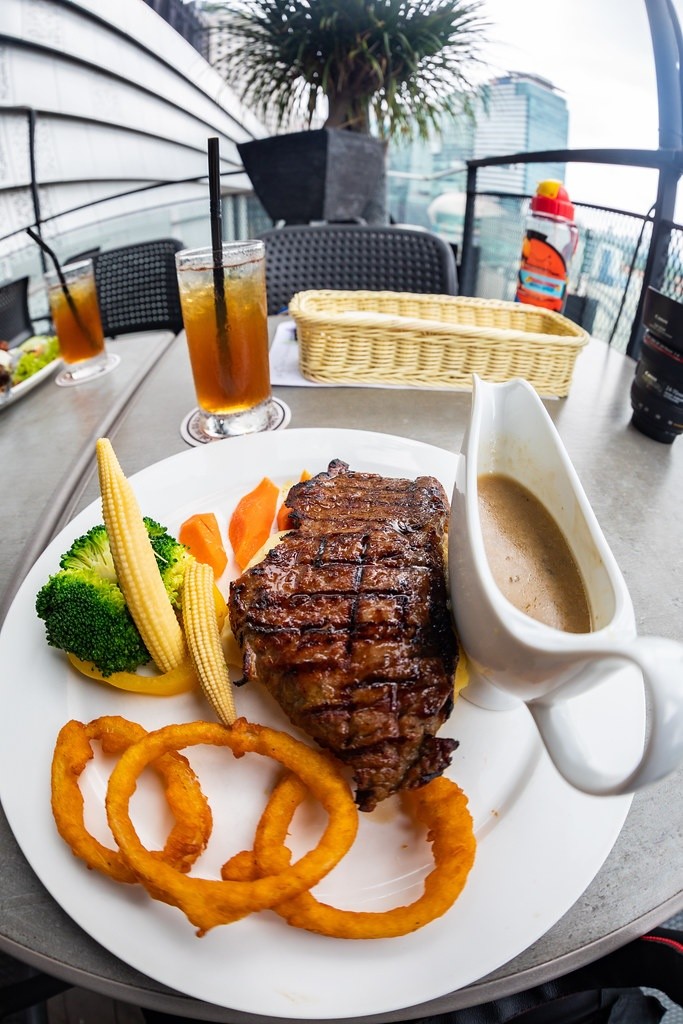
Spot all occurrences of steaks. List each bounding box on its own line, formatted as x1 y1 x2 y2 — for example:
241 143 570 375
228 455 458 810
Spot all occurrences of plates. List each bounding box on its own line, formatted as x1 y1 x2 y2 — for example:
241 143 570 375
0 426 645 1019
0 346 63 411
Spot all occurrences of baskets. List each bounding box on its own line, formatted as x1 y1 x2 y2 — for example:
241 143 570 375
289 289 590 397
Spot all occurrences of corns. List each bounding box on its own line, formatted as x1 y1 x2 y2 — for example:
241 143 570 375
180 563 236 726
96 438 180 671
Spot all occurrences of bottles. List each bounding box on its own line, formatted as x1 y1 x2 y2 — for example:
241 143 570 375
514 178 579 313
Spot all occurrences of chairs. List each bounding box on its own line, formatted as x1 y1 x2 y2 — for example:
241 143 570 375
65 238 186 337
255 224 459 315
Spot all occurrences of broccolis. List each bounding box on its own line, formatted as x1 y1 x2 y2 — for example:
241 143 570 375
35 516 188 677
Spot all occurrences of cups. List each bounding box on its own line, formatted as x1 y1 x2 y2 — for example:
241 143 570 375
175 239 275 438
44 258 108 379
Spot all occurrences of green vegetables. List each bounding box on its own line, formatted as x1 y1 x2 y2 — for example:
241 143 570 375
14 338 59 383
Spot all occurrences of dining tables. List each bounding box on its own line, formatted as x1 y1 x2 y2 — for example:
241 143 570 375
0 314 683 1024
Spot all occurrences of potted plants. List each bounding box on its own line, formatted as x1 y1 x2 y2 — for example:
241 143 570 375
193 0 494 226
